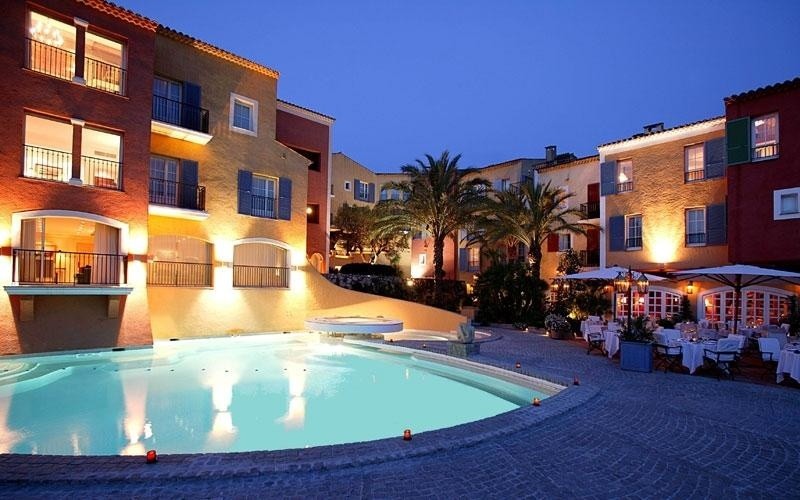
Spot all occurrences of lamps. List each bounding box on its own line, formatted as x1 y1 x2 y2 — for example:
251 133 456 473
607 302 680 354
215 261 233 268
291 265 305 272
0 246 12 256
659 263 665 270
128 254 148 263
686 280 693 294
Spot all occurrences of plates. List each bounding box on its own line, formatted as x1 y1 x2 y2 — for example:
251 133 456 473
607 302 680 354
788 349 798 351
705 340 716 346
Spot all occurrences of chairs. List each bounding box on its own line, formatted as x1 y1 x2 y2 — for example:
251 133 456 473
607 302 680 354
77 267 91 284
581 314 800 387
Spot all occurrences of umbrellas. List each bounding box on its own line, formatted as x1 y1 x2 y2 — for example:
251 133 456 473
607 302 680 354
548 263 670 322
667 260 799 334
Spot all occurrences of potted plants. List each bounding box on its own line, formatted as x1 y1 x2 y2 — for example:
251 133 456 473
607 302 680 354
546 314 571 339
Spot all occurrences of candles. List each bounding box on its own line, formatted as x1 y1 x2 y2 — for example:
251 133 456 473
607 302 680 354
574 376 579 385
534 397 539 405
516 362 521 368
147 450 156 463
404 429 411 440
